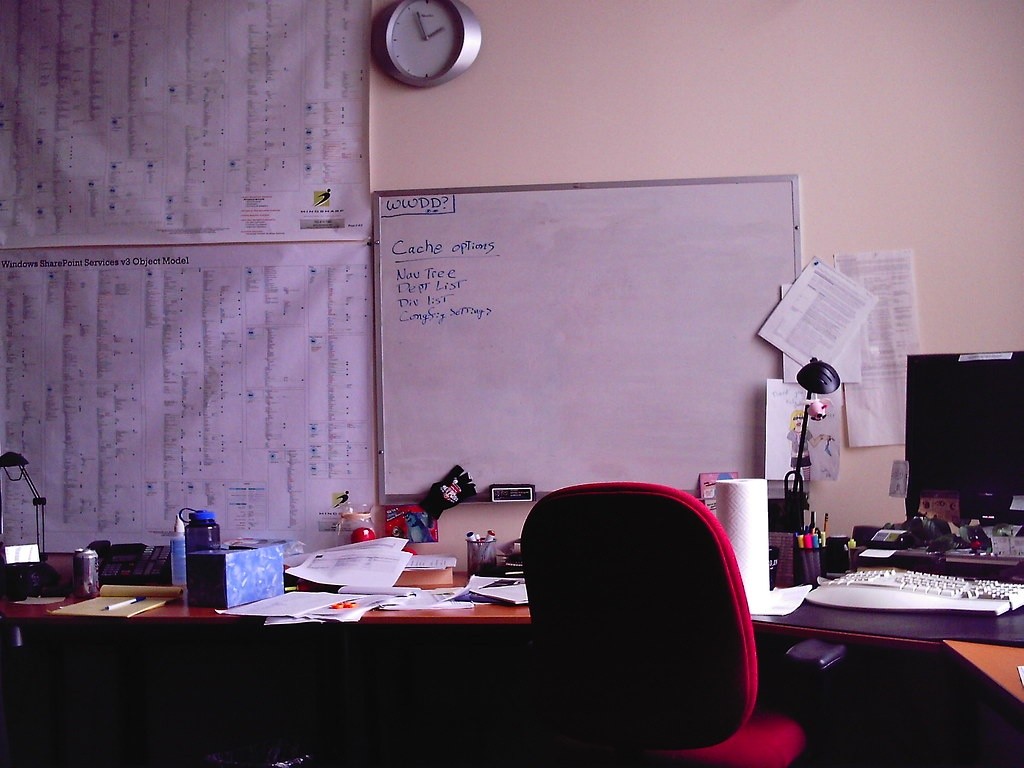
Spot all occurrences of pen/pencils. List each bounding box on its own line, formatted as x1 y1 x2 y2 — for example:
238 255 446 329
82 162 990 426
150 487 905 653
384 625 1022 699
105 595 147 612
795 510 831 582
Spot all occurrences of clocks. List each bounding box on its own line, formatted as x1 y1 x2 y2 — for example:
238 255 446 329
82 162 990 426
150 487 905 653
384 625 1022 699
377 0 482 88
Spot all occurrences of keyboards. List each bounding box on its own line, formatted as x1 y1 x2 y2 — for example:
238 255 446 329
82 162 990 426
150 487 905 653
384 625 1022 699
805 569 1024 615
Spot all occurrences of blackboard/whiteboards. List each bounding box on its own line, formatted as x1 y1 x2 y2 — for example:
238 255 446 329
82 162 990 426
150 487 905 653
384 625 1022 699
366 169 807 511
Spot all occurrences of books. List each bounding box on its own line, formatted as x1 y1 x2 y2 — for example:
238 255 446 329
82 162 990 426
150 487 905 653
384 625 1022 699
470 583 528 605
44 585 184 618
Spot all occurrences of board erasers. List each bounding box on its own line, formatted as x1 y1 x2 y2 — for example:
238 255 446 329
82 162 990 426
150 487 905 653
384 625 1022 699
488 483 536 503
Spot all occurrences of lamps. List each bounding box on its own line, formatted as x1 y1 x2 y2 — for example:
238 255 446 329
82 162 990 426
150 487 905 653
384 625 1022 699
783 356 843 530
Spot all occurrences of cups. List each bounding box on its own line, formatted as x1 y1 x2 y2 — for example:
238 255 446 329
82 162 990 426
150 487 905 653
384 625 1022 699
6 563 40 601
466 538 496 580
769 546 778 591
793 544 826 590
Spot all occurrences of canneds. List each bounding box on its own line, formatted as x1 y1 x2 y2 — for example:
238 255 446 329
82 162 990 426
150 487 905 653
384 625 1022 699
73 548 99 598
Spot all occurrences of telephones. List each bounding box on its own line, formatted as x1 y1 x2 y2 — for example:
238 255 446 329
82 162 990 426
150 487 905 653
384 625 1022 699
79 537 172 586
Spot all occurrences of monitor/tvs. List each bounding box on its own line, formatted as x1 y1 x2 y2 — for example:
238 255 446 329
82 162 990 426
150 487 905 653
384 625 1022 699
905 351 1024 527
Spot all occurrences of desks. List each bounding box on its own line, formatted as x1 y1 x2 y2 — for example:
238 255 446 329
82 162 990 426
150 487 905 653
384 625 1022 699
0 571 1024 768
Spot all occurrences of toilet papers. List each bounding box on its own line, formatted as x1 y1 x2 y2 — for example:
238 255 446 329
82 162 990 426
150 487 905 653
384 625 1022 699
715 479 771 605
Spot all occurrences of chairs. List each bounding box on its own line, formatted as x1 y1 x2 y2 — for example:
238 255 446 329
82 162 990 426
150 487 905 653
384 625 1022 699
520 481 805 768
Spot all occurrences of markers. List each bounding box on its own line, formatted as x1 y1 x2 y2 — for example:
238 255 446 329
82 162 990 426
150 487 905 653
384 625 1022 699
465 530 501 580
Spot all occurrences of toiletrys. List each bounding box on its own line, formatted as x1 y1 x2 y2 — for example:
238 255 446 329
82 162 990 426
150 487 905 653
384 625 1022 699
170 514 187 587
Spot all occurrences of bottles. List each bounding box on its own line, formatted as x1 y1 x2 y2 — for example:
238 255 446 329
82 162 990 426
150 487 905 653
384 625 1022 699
179 508 221 592
171 514 187 586
335 503 375 548
